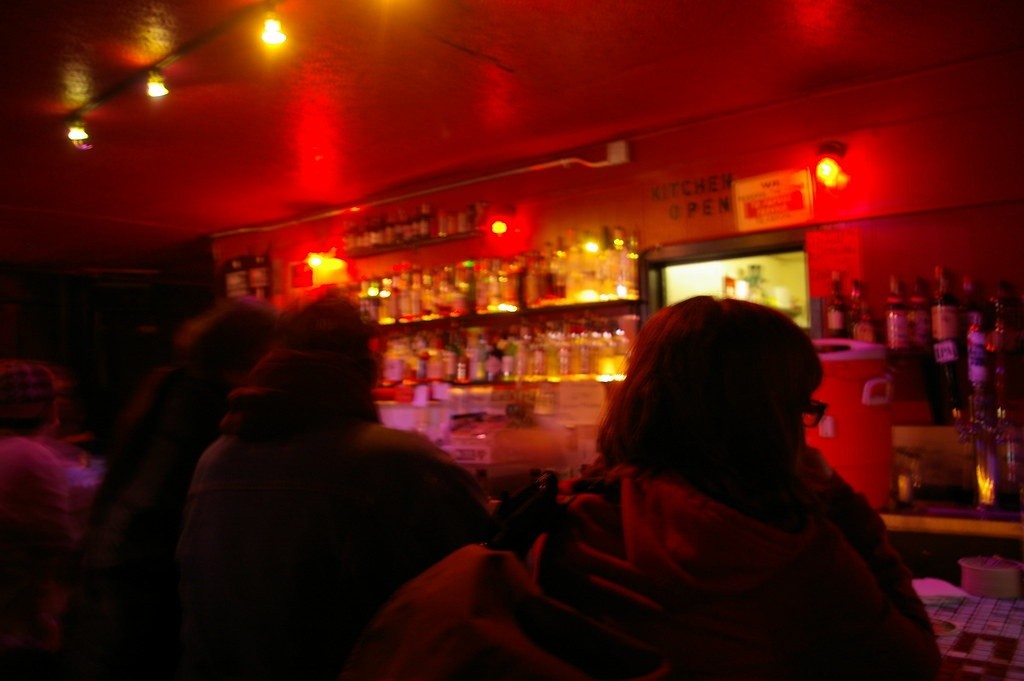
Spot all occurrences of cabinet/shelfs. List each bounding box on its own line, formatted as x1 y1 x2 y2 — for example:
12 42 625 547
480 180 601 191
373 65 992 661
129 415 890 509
370 296 641 388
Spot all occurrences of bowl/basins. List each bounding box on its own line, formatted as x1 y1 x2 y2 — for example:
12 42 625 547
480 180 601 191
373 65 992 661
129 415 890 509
959 557 1024 599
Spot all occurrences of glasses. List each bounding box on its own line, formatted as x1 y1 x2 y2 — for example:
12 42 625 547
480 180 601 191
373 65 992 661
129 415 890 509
801 400 827 427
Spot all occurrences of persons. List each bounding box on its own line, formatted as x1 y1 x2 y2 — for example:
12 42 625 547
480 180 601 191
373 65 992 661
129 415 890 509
112 294 500 681
0 355 109 681
543 297 939 680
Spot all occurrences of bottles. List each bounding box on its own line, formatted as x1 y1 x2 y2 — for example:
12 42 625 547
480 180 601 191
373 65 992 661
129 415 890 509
249 255 271 299
224 255 248 298
343 202 641 386
884 264 1024 513
825 276 875 350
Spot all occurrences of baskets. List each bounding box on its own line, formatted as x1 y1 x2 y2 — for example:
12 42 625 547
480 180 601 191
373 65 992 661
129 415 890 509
957 556 1024 600
